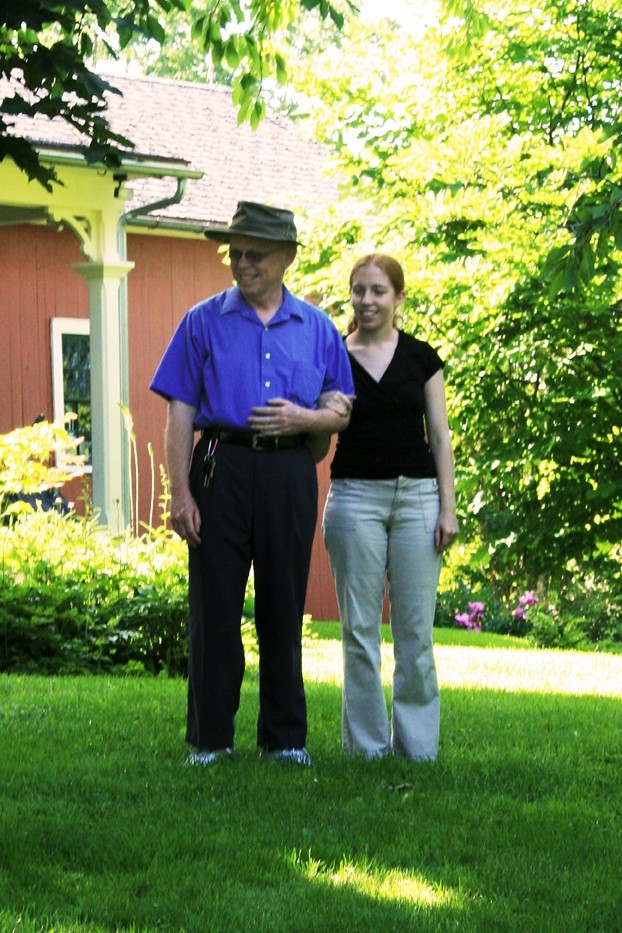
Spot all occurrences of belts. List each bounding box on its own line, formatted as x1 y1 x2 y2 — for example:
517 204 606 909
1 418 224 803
200 428 309 452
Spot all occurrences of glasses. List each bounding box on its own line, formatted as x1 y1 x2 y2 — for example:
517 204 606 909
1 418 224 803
227 248 284 264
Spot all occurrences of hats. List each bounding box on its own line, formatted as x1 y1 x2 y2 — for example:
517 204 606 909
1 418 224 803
202 200 307 249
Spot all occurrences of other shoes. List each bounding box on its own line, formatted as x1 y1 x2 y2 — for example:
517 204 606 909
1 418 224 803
258 744 311 767
182 744 235 769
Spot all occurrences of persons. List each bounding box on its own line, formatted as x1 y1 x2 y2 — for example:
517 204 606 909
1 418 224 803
317 254 460 762
150 201 356 764
